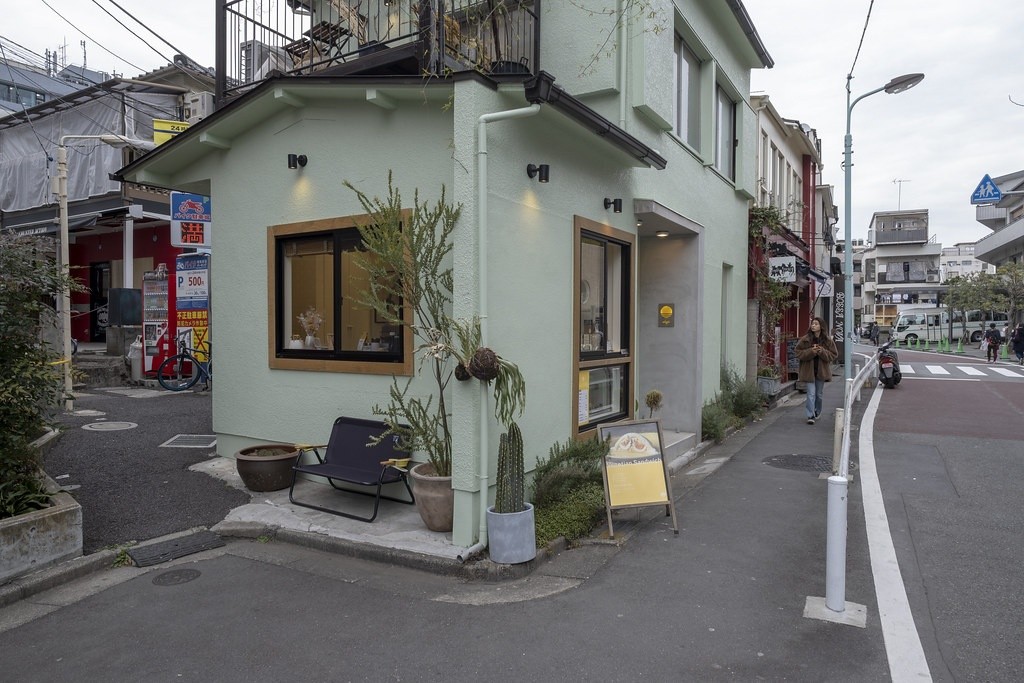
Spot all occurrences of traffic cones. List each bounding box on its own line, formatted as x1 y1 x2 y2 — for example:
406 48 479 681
894 337 902 349
956 338 964 353
999 342 1010 359
915 338 923 351
924 337 932 351
944 337 951 352
937 339 943 352
906 337 913 350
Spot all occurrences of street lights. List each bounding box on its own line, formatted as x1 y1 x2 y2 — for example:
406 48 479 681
830 0 926 483
56 133 129 412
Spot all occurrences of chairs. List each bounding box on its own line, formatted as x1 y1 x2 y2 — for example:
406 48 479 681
286 415 417 523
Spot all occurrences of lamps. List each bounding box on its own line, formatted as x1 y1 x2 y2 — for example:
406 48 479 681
526 163 549 183
604 198 622 213
287 153 307 170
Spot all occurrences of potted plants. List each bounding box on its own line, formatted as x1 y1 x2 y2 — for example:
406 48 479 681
398 0 533 115
234 443 300 493
484 417 543 565
329 162 530 541
757 366 783 395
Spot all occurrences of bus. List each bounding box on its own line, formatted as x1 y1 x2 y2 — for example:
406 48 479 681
889 309 1012 344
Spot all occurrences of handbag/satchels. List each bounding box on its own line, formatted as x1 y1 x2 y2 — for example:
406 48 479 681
979 337 989 351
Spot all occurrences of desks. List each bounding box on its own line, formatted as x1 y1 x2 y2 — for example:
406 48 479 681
303 19 354 72
281 36 329 74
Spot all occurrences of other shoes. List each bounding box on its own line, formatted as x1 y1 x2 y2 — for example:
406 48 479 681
856 343 879 347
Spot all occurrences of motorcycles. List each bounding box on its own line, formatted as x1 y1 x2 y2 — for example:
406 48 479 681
877 338 903 388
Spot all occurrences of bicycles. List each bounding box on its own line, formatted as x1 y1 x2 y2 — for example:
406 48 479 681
155 327 212 395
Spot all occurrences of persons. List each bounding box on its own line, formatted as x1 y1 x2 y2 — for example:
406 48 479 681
1003 323 1009 345
794 317 838 424
852 318 897 346
985 323 1002 363
1010 322 1024 365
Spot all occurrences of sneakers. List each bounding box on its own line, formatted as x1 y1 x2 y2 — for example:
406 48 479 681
815 411 822 419
986 358 1023 365
807 416 815 424
